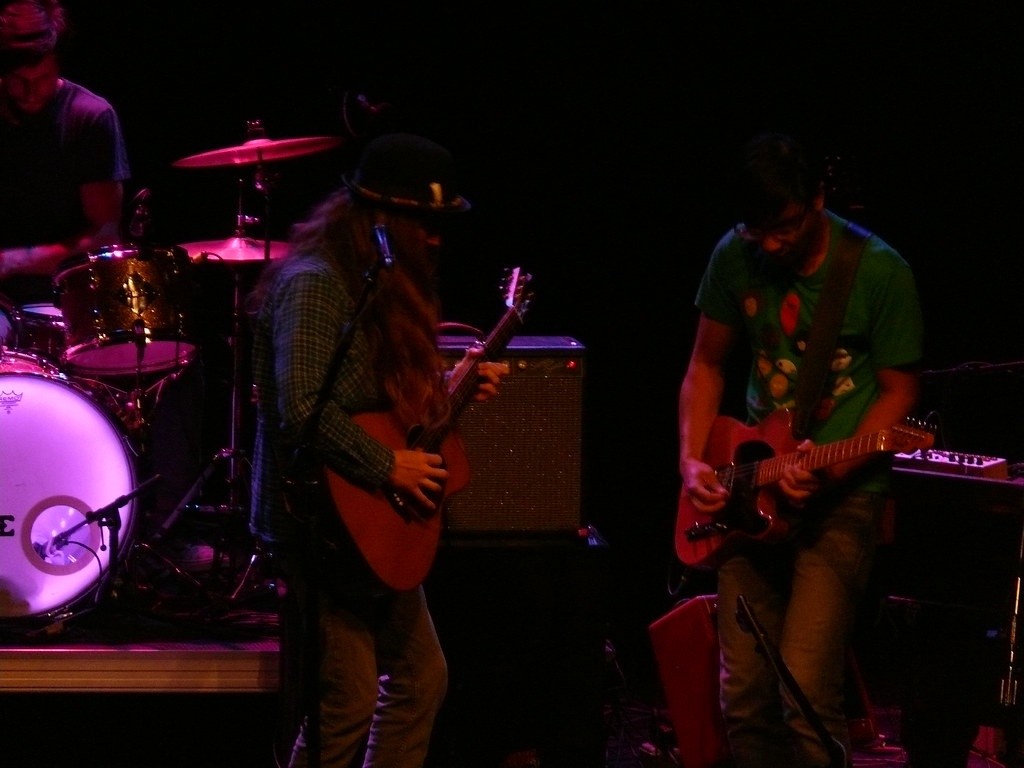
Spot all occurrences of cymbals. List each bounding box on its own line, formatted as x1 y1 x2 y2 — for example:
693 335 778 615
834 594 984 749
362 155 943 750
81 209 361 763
172 120 339 167
175 237 289 261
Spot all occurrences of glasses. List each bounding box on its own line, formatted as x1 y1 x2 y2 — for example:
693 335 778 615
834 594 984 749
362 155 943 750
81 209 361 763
734 200 812 241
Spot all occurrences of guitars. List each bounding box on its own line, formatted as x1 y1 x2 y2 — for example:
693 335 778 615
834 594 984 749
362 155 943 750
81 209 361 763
283 267 536 606
675 406 936 571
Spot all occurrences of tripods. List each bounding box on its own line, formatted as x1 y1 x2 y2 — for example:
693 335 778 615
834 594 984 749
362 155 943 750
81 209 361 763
128 265 259 619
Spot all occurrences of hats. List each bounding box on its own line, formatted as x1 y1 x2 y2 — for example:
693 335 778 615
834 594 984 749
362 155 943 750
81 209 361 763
340 133 472 214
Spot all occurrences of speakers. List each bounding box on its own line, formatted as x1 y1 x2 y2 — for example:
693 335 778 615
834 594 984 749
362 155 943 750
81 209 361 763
847 467 1024 768
435 333 586 545
648 593 731 768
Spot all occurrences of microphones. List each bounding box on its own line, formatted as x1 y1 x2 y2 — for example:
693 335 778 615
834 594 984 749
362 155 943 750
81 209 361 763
128 196 151 239
348 92 392 137
133 271 156 297
134 319 146 363
605 645 632 692
373 223 393 269
190 252 210 267
34 539 69 560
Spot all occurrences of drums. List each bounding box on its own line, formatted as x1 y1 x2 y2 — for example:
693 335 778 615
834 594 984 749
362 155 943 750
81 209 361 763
22 302 66 361
0 350 140 635
49 243 202 375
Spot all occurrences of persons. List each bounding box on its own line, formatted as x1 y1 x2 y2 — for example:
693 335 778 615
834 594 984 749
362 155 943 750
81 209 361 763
679 130 922 767
252 134 505 768
0 0 130 295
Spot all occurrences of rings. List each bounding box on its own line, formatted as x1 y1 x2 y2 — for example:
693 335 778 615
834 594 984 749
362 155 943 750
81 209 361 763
688 493 693 499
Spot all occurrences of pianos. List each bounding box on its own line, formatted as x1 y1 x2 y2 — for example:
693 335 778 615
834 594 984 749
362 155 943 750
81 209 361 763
896 449 1022 488
